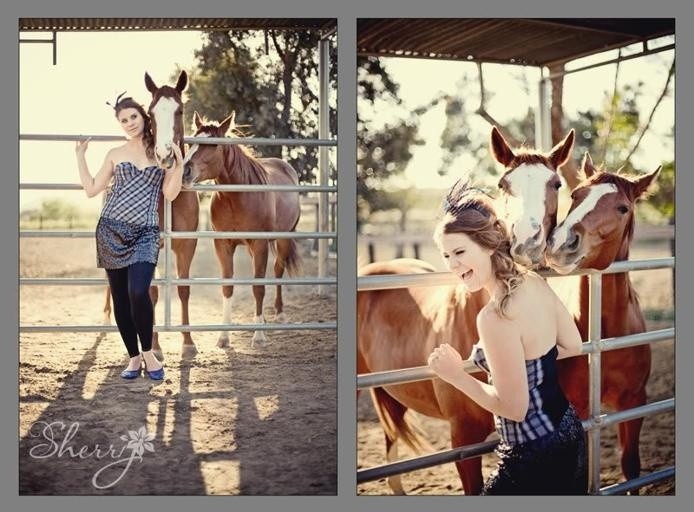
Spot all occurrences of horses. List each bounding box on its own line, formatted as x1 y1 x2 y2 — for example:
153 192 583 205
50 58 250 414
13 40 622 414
543 151 662 495
356 126 575 496
102 69 200 362
182 110 303 349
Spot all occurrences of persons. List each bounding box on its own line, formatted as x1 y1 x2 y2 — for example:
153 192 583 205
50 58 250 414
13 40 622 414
74 96 185 381
423 196 594 494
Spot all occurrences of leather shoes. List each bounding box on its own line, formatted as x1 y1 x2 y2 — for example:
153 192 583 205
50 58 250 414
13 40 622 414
120 351 165 380
